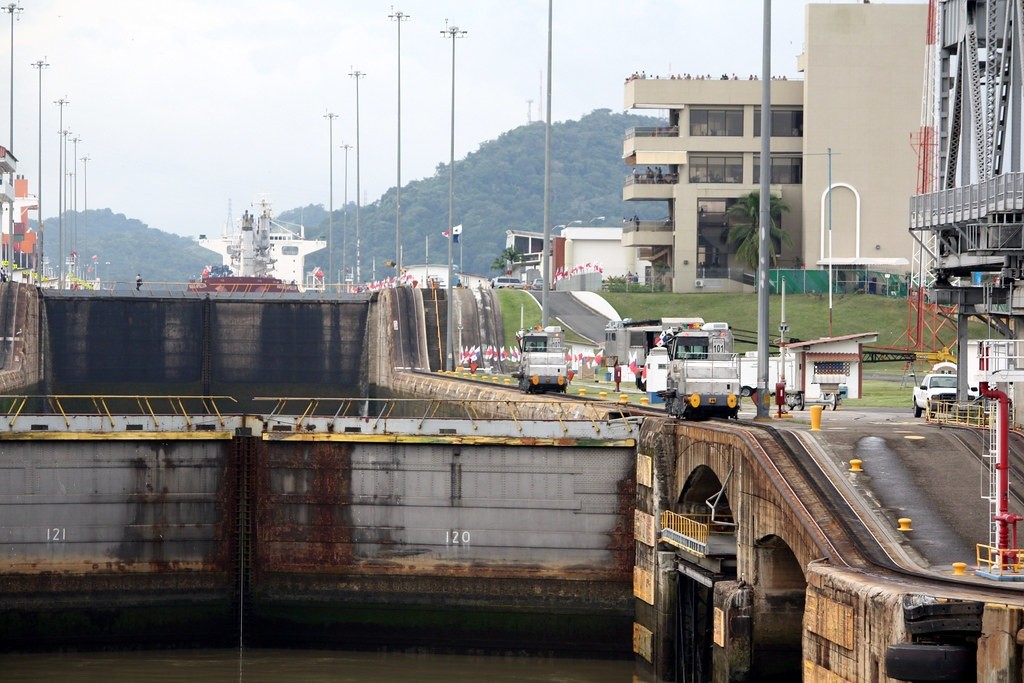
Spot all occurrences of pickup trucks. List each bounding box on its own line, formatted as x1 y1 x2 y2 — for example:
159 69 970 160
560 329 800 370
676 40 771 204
911 374 958 418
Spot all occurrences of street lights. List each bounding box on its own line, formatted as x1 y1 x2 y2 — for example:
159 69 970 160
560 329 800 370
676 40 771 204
0 0 24 284
338 139 353 292
66 169 75 276
67 134 83 279
387 3 412 290
438 17 467 374
79 153 92 282
29 54 51 288
347 64 367 284
322 109 339 293
52 94 70 291
57 127 72 284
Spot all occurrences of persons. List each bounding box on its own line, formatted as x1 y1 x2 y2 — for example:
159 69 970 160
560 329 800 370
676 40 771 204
477 279 482 288
682 346 694 358
427 276 432 288
456 279 462 287
623 214 640 232
690 169 722 183
665 216 672 224
492 279 494 289
1 267 8 282
633 166 663 184
622 270 639 283
625 70 787 85
699 208 704 217
135 274 143 291
209 273 212 277
612 358 622 392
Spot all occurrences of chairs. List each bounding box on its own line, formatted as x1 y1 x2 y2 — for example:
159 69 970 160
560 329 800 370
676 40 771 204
952 380 957 388
931 380 940 386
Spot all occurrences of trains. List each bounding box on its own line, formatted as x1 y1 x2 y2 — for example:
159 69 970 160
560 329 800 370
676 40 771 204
645 323 742 423
512 326 570 395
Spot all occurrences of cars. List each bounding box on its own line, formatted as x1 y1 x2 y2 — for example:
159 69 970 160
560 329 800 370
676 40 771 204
530 278 543 290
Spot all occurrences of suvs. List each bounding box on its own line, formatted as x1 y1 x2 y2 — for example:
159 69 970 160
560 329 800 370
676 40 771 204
492 276 526 290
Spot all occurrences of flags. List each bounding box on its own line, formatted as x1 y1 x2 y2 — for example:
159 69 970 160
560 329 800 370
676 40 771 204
368 273 413 290
554 261 603 283
443 224 462 243
312 267 324 279
574 348 603 369
459 345 521 364
655 327 675 347
628 350 647 383
566 349 572 364
